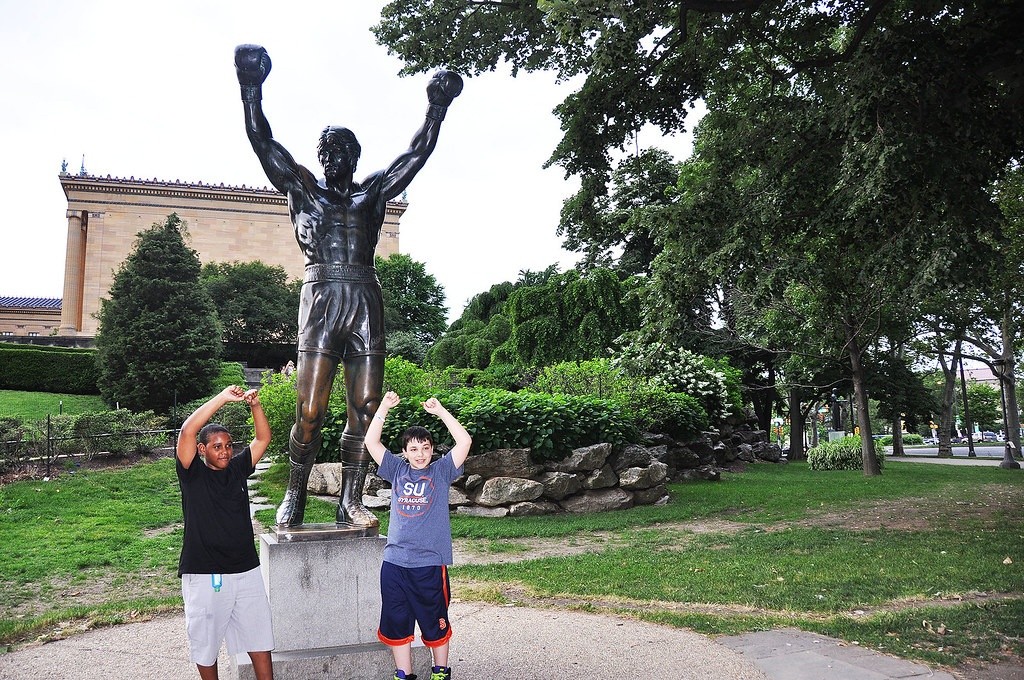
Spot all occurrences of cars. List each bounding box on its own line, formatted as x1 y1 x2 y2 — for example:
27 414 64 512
922 430 1005 445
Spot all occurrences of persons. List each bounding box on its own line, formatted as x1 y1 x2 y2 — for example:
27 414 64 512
235 44 464 527
176 385 275 680
365 391 472 680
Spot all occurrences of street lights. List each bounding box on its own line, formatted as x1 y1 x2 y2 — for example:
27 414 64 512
832 387 855 434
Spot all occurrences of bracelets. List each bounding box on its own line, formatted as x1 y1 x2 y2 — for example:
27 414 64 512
375 414 386 422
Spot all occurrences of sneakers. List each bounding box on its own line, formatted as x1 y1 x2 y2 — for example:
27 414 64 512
430 666 452 680
393 669 418 680
